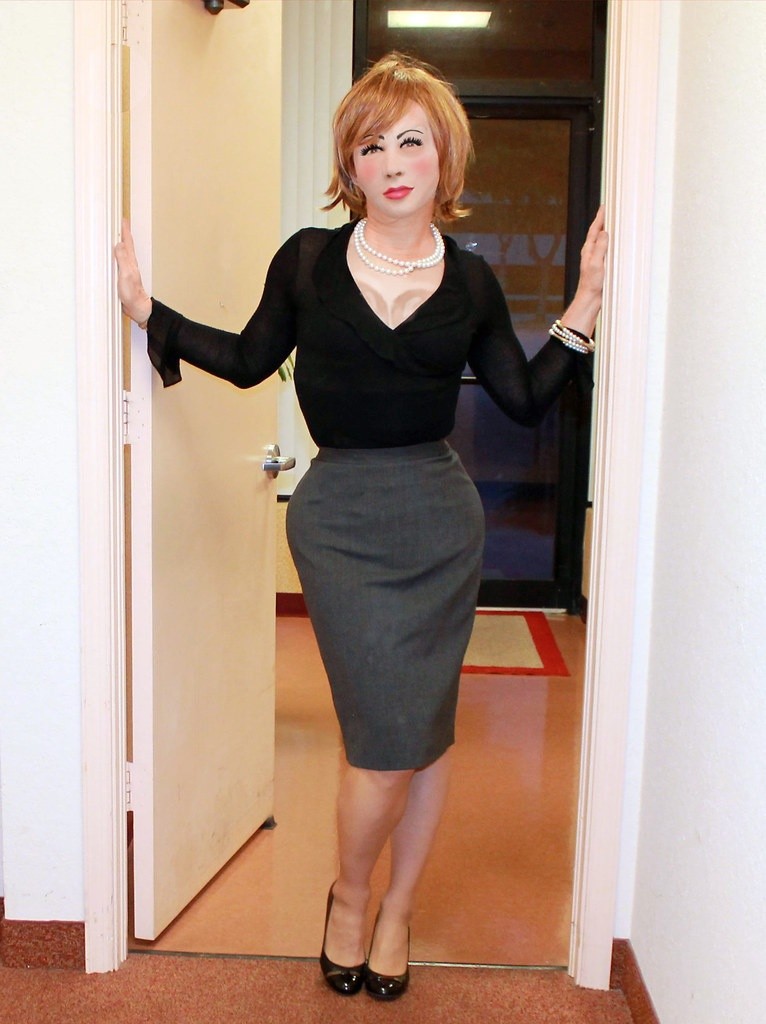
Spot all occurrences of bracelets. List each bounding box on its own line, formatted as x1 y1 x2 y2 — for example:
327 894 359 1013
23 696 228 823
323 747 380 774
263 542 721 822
548 319 595 353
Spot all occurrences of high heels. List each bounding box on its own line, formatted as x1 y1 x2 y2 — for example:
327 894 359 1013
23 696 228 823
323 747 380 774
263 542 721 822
319 878 367 994
365 901 411 1000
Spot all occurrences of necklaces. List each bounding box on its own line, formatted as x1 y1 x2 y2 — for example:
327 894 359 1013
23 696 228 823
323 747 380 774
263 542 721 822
354 218 446 277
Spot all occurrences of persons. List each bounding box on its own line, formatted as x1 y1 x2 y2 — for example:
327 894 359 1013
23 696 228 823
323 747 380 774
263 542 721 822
112 53 607 1001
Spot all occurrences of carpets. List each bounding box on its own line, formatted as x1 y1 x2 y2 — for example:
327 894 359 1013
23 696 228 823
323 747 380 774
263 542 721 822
458 609 572 678
